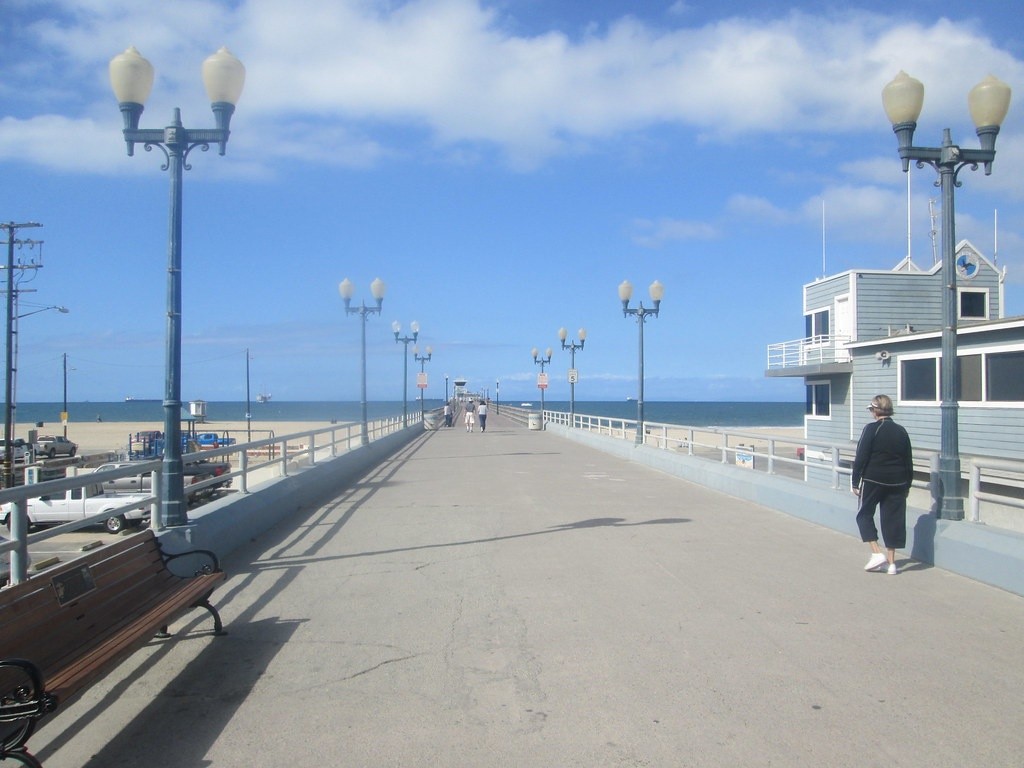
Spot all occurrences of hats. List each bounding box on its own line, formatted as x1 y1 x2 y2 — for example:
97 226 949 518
468 398 474 402
866 401 894 412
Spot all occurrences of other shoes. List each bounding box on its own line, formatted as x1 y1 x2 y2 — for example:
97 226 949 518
864 553 887 571
880 563 897 574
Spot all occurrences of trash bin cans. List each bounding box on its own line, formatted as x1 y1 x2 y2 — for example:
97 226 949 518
424 412 439 431
528 413 543 431
330 418 338 424
35 421 43 427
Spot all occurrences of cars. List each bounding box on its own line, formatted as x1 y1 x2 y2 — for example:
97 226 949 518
0 438 28 463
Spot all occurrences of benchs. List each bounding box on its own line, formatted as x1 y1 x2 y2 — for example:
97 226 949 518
0 528 226 767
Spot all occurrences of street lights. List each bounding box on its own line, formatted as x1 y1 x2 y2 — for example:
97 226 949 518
452 383 456 401
558 326 587 426
443 372 450 405
391 317 420 428
618 278 664 446
531 346 552 412
496 378 500 414
880 67 1016 518
108 45 247 528
412 344 433 419
338 274 386 445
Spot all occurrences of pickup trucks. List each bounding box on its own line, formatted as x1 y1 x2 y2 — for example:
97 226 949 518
197 433 236 446
37 434 77 458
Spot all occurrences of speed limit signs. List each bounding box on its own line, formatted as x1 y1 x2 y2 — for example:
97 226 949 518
568 369 579 383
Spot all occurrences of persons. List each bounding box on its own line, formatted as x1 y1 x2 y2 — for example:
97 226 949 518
464 399 476 433
852 394 914 576
477 401 488 432
443 402 453 427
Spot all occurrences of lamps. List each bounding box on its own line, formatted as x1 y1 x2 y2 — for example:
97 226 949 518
875 350 889 361
